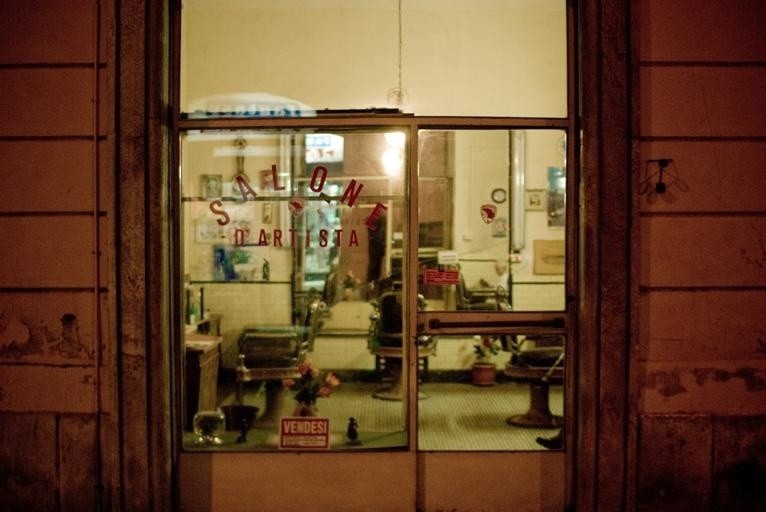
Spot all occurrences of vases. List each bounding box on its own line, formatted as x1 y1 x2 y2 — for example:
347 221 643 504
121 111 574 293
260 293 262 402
293 399 318 418
473 363 496 386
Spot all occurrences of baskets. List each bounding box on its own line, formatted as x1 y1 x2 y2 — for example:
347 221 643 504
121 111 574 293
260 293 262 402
423 270 459 286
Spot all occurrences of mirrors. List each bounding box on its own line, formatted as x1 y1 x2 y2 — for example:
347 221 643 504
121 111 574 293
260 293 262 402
291 266 382 331
417 264 564 312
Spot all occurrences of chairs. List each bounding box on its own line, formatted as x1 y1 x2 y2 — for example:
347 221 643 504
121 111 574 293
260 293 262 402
504 334 566 429
243 300 321 353
369 293 435 402
237 332 304 425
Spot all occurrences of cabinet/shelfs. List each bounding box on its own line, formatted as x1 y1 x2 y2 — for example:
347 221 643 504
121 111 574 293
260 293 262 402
385 199 450 311
184 314 223 431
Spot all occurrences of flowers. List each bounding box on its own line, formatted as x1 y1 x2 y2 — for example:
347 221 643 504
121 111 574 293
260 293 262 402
284 359 339 400
473 335 500 359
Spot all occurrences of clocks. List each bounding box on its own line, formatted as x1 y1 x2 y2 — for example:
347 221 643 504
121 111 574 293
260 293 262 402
492 189 506 203
